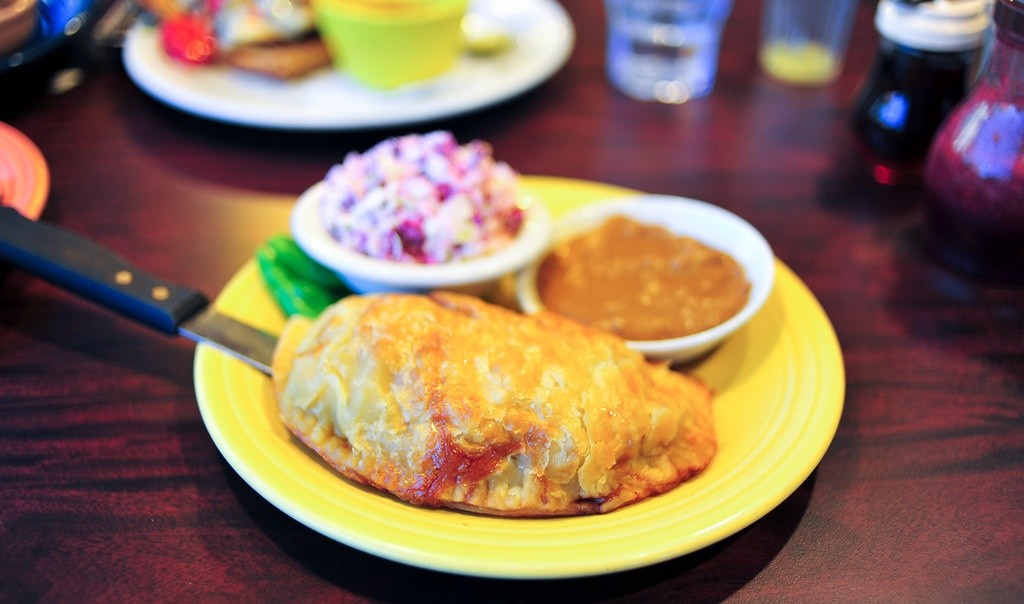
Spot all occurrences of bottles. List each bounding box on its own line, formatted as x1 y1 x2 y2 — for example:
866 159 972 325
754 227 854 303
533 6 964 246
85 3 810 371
860 1 1023 241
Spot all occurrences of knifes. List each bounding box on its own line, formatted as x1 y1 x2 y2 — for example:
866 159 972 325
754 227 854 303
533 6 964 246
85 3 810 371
3 200 273 374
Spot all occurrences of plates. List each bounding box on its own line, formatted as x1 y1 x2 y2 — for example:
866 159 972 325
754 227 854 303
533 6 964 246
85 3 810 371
118 3 574 130
195 173 852 585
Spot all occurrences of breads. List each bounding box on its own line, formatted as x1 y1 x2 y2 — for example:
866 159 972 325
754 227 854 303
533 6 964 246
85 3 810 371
275 292 716 519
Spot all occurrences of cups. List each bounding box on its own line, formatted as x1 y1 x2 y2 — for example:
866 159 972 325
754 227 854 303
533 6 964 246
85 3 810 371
760 1 849 87
603 0 729 102
